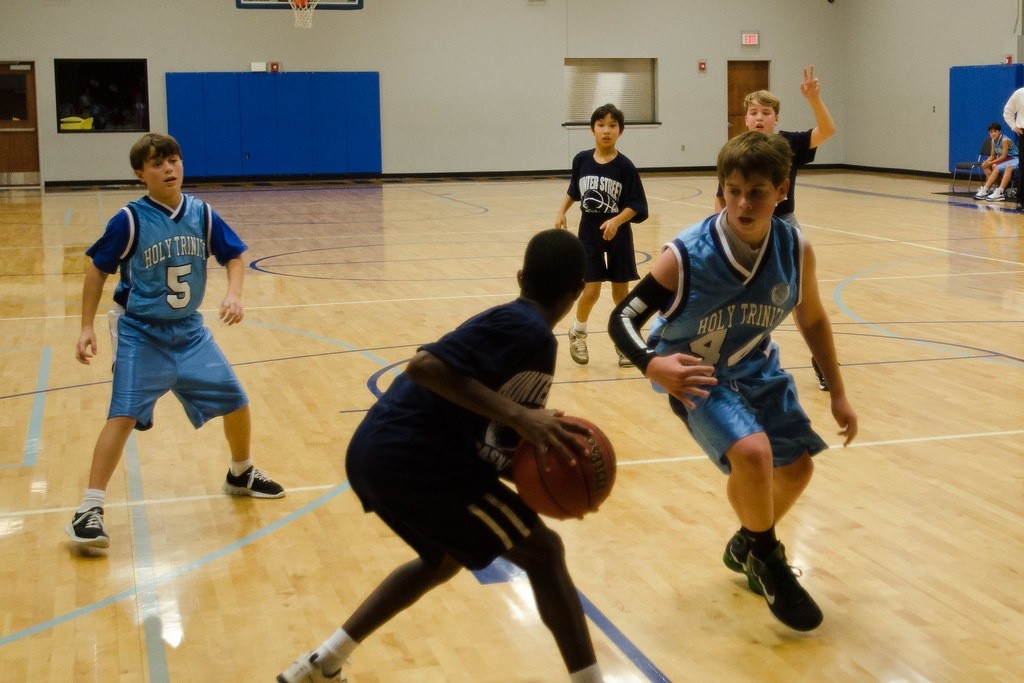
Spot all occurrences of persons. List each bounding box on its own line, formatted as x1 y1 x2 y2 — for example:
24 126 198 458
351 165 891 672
973 86 1024 211
277 229 639 683
64 133 287 549
554 104 649 369
715 64 836 233
608 129 859 634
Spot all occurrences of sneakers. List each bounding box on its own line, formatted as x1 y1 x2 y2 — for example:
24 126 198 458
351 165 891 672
276 651 349 683
723 531 764 595
985 188 1005 201
973 186 992 199
64 506 110 548
614 345 636 368
811 357 829 390
747 540 823 632
223 465 285 498
568 327 589 364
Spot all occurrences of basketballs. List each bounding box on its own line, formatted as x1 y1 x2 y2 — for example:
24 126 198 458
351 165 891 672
511 416 618 522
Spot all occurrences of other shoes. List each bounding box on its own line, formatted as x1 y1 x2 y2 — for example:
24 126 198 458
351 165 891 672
1016 203 1024 210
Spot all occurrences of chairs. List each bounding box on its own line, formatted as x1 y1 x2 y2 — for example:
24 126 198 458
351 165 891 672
998 141 1019 200
952 138 991 194
